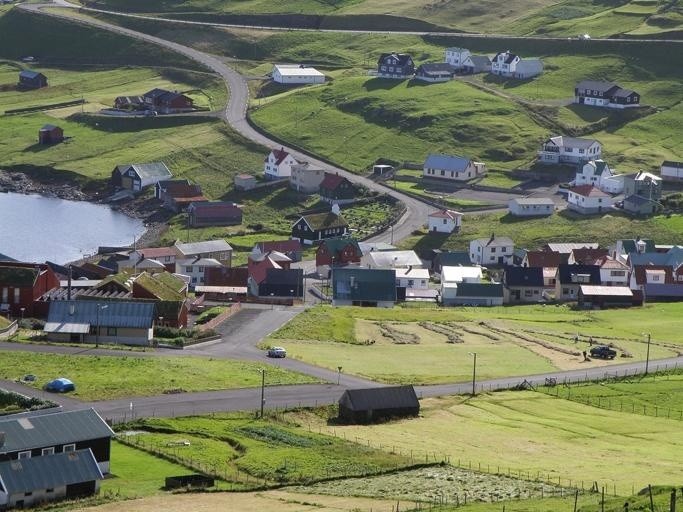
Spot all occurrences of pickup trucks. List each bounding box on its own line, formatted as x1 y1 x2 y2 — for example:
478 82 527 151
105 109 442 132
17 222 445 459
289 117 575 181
590 346 617 361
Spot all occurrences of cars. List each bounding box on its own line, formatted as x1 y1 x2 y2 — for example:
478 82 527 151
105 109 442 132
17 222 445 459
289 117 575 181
268 346 286 359
42 378 75 395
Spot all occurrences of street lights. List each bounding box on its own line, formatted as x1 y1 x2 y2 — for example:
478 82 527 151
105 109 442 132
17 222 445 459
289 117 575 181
467 351 477 397
640 331 652 378
255 368 267 419
